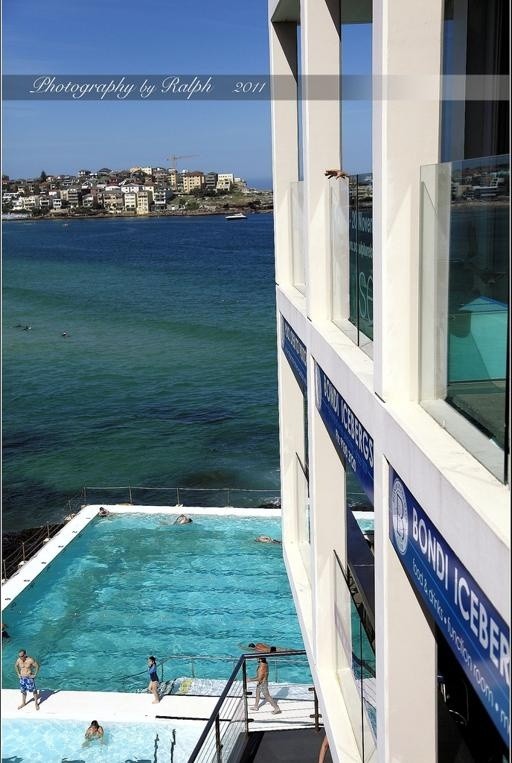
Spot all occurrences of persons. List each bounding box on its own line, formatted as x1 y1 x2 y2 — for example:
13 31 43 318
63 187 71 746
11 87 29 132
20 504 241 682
59 331 73 338
318 733 331 762
238 640 304 655
147 655 161 703
14 648 40 710
98 506 110 517
173 513 193 524
254 535 283 545
21 323 30 332
246 656 281 713
1 619 11 638
81 719 107 750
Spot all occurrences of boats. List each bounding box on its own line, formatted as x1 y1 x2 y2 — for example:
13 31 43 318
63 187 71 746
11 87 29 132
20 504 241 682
225 212 247 219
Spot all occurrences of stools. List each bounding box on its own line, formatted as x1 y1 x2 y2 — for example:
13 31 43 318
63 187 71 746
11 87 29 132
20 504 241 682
438 656 472 729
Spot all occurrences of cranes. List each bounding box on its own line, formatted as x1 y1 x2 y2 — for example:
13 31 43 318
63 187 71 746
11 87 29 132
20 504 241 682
166 153 197 168
